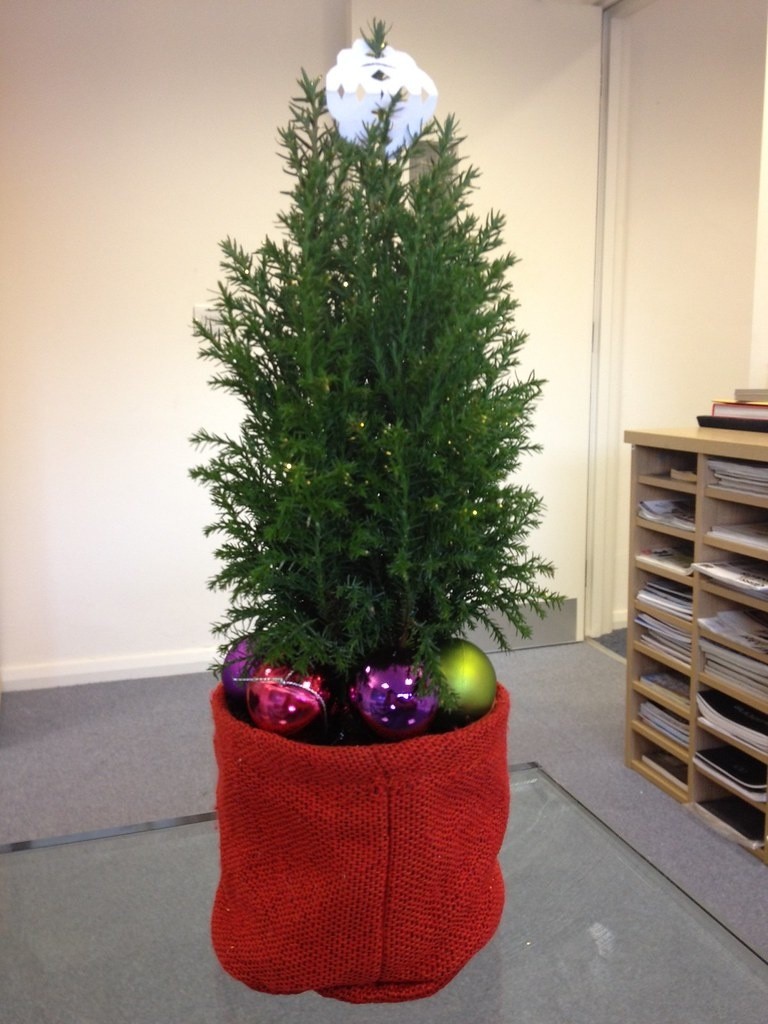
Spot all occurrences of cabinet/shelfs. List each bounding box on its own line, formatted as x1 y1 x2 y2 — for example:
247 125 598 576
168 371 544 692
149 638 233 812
620 381 768 869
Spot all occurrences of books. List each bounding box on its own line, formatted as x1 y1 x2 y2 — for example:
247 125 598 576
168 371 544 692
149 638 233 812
632 388 767 851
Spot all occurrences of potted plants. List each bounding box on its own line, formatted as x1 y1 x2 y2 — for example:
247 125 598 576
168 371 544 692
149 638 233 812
192 70 551 1005
